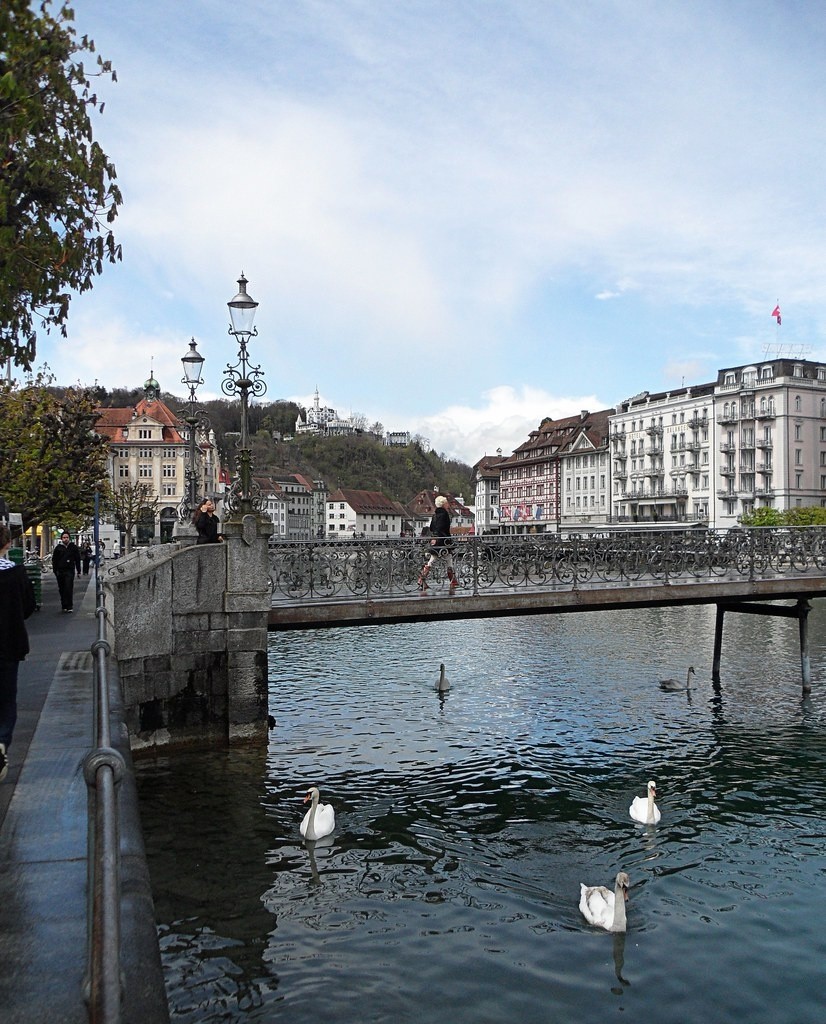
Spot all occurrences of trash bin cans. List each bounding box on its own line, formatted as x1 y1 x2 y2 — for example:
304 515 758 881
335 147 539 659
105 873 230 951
25 564 43 611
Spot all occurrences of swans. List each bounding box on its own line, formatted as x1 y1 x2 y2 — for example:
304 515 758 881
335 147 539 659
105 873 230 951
434 663 451 691
579 872 630 932
299 787 335 840
659 666 697 689
629 780 662 825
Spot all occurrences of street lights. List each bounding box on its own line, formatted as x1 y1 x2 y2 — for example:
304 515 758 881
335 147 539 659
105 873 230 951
178 335 207 513
222 274 276 526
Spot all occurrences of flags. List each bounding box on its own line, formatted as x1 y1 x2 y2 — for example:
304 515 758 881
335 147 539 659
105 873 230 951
769 298 784 326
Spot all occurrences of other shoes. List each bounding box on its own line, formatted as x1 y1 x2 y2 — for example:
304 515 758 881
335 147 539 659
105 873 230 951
63 608 74 613
0 742 9 780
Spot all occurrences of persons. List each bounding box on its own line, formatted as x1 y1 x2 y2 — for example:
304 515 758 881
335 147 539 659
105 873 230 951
415 494 466 597
0 525 36 786
193 496 225 546
52 523 123 617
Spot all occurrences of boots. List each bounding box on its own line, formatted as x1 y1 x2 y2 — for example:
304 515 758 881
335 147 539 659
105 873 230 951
417 565 430 589
447 570 463 588
